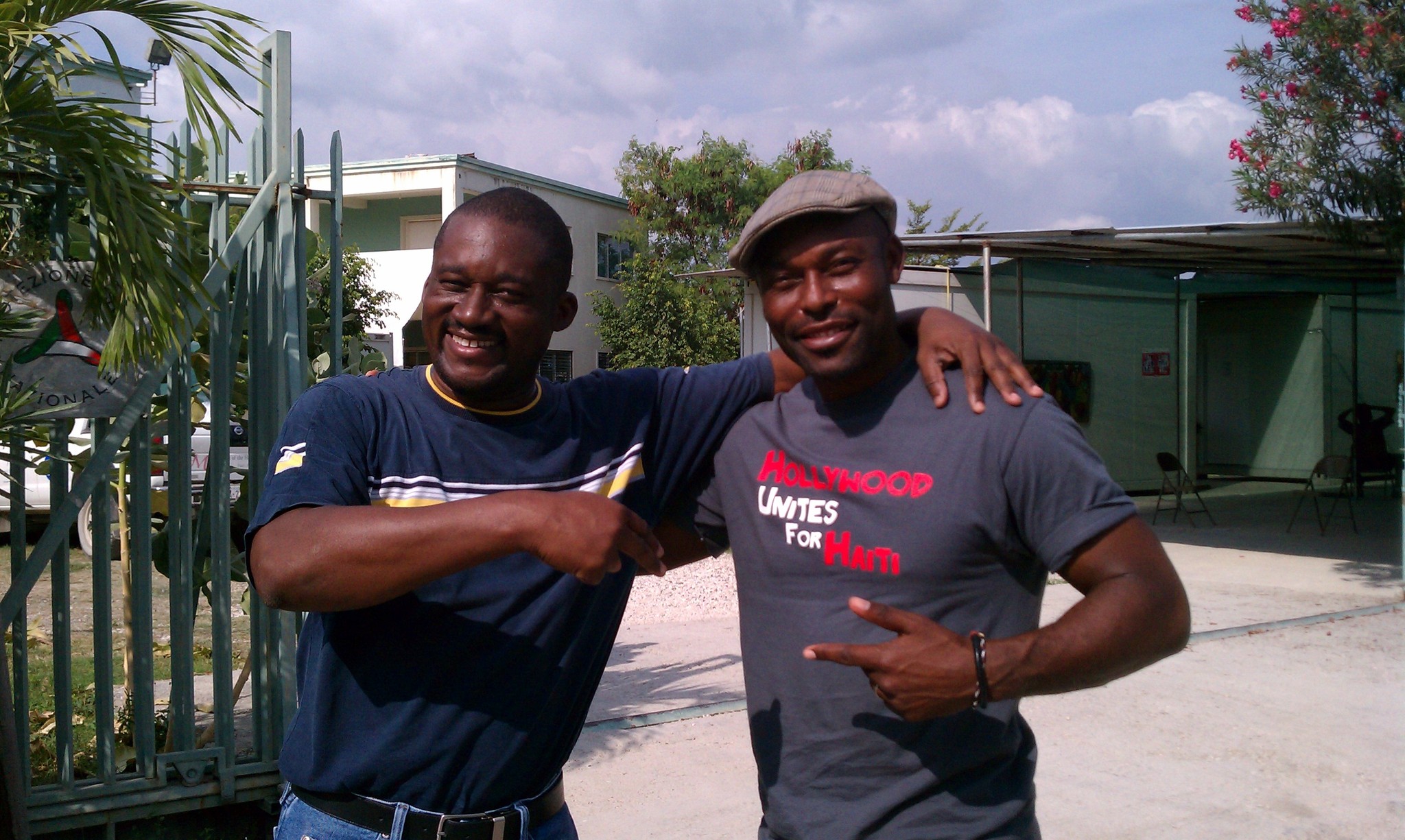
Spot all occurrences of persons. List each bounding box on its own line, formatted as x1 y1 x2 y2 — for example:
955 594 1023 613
243 190 1047 840
635 167 1194 840
1335 403 1404 500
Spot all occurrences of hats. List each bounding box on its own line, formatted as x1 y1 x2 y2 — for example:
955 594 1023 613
727 168 897 283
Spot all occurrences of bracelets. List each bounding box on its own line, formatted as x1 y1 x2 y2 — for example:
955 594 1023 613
967 630 992 712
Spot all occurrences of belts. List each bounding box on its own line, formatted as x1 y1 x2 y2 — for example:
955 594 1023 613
289 768 565 840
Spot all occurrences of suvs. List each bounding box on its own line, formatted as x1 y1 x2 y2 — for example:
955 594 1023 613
0 388 249 544
